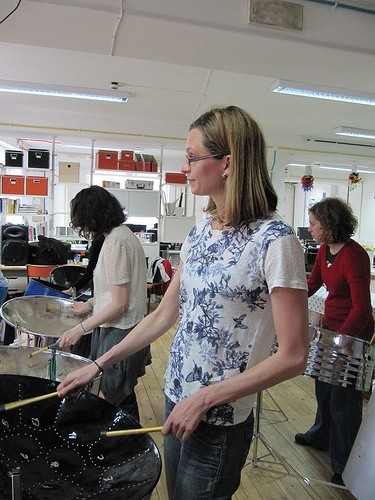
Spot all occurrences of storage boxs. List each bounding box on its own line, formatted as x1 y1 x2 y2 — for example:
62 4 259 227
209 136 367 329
25 177 48 195
166 172 187 185
1 174 24 194
95 149 158 171
27 148 50 169
4 150 24 166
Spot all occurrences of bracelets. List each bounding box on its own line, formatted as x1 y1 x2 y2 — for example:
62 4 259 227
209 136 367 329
94 361 104 373
81 323 88 334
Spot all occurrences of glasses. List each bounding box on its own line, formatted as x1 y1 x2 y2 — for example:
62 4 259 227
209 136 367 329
184 150 229 166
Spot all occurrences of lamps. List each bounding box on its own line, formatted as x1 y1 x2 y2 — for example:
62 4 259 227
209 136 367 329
0 78 131 104
334 126 375 140
270 79 375 106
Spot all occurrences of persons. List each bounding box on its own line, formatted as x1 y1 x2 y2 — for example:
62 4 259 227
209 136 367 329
56 186 153 423
294 198 375 487
57 106 310 500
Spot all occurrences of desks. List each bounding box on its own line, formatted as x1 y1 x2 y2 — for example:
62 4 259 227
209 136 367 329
0 264 26 278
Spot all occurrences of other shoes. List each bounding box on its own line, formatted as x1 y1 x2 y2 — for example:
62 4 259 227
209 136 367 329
296 432 306 442
333 472 345 486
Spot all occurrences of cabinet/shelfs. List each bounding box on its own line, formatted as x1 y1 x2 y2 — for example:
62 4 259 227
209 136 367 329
91 139 161 257
156 182 196 244
0 136 56 238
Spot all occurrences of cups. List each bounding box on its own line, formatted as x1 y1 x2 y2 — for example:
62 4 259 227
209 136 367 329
67 260 73 265
83 258 88 265
74 257 79 264
135 232 151 243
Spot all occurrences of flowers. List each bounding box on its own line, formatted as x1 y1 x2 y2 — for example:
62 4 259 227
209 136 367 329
345 173 363 191
301 176 314 193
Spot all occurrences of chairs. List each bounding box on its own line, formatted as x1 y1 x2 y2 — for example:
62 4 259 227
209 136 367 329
27 263 59 283
146 258 172 314
